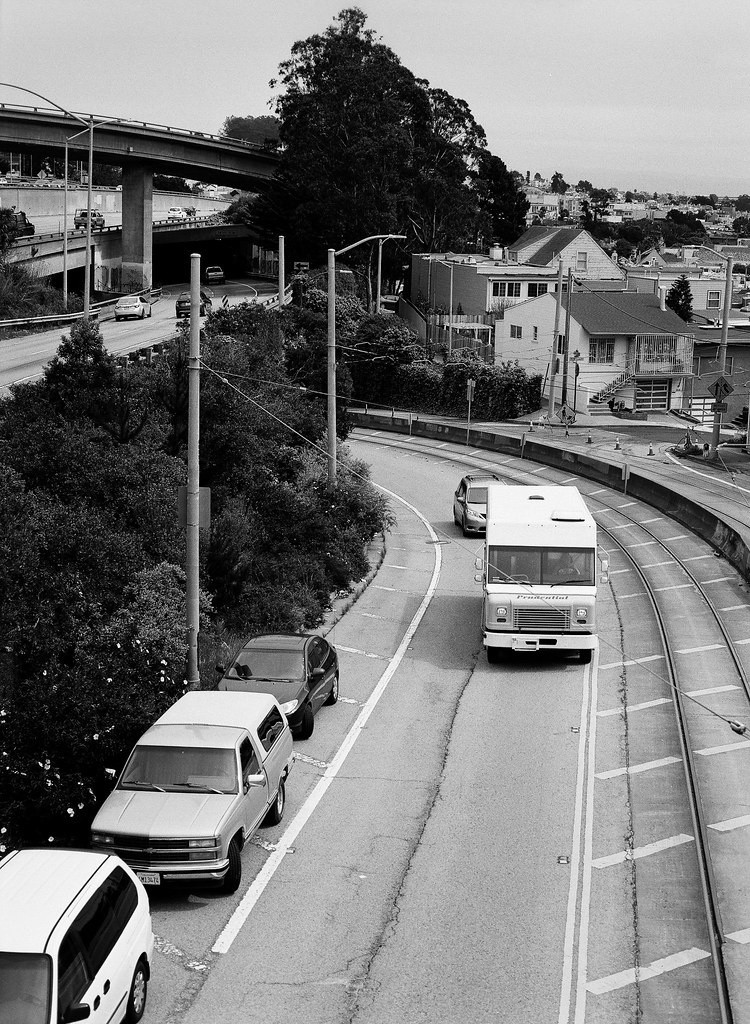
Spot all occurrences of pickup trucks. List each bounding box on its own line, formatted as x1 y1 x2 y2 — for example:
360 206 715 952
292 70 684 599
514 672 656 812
206 266 225 284
74 207 105 231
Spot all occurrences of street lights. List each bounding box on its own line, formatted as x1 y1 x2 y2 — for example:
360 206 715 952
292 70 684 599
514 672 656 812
298 270 355 366
681 243 734 462
326 233 407 487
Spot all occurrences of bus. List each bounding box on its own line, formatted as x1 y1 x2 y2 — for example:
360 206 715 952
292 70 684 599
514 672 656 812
474 484 610 663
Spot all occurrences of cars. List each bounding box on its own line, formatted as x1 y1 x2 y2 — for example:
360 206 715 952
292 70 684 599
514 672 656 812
114 296 152 321
214 632 340 741
175 290 213 319
184 206 197 216
168 207 187 219
0 176 65 189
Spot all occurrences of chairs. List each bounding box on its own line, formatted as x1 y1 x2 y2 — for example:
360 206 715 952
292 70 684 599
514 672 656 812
155 748 193 785
196 751 236 777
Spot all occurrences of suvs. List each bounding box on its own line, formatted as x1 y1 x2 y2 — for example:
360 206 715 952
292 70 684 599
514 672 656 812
453 475 510 539
0 850 154 1024
90 690 295 899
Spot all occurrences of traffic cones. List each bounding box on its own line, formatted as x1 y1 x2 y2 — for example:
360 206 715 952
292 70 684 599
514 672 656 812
585 430 594 444
646 441 656 457
613 436 622 451
528 419 536 432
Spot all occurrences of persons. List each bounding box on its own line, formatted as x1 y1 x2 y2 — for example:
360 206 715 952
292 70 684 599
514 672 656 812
552 554 579 575
